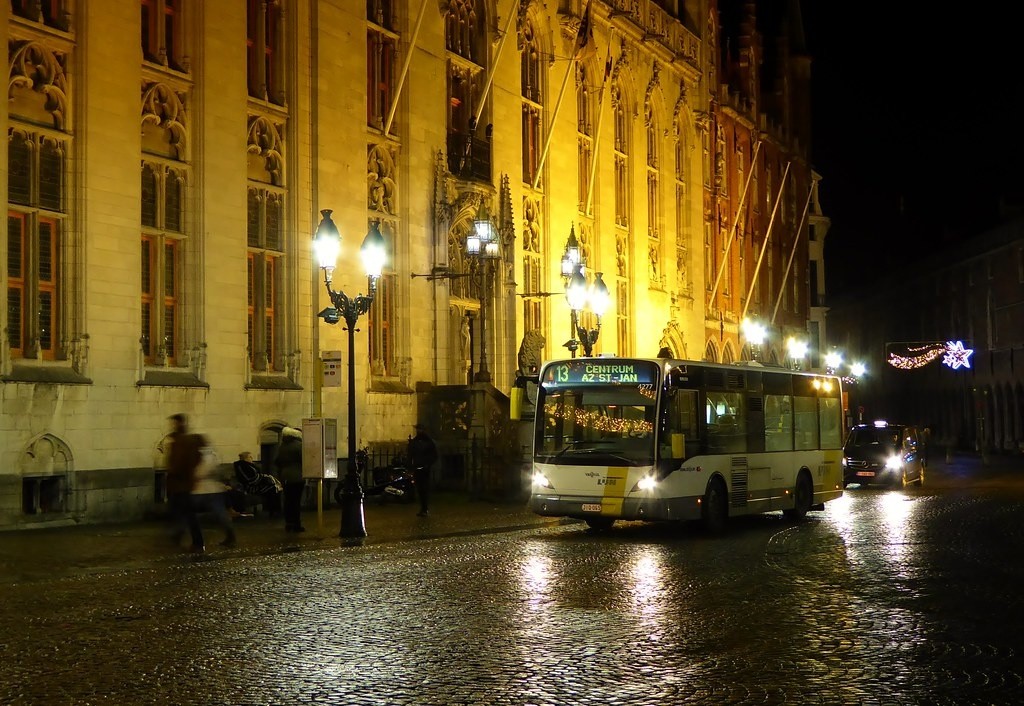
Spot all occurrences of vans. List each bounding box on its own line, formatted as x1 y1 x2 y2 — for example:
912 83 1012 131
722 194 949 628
842 424 926 491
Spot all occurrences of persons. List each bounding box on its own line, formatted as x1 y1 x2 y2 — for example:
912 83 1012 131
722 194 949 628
159 413 206 548
921 428 930 443
192 432 239 548
890 433 898 443
406 425 438 518
233 450 283 520
275 426 308 533
718 415 734 432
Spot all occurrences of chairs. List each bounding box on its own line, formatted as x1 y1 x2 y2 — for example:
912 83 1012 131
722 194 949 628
718 415 735 433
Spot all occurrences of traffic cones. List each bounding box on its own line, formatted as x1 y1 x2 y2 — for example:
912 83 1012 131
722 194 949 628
982 445 993 468
944 446 953 466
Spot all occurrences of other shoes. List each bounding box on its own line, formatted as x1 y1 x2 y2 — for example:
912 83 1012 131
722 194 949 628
285 524 305 533
417 508 428 517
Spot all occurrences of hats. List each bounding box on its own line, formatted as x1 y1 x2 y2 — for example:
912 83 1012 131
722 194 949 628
415 424 424 429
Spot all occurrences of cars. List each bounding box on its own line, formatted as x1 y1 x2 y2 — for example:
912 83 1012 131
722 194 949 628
938 421 977 453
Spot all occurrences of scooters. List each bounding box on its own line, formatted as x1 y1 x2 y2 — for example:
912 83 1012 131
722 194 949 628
333 448 415 505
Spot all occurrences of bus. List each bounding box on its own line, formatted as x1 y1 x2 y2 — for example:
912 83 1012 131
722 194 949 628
510 349 845 533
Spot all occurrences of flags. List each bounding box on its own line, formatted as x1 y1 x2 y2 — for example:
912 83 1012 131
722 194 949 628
519 0 531 27
575 1 597 59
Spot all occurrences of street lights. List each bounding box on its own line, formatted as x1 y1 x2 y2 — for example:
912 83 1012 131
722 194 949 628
557 228 585 357
465 193 498 383
970 384 987 451
567 264 606 356
311 208 389 547
